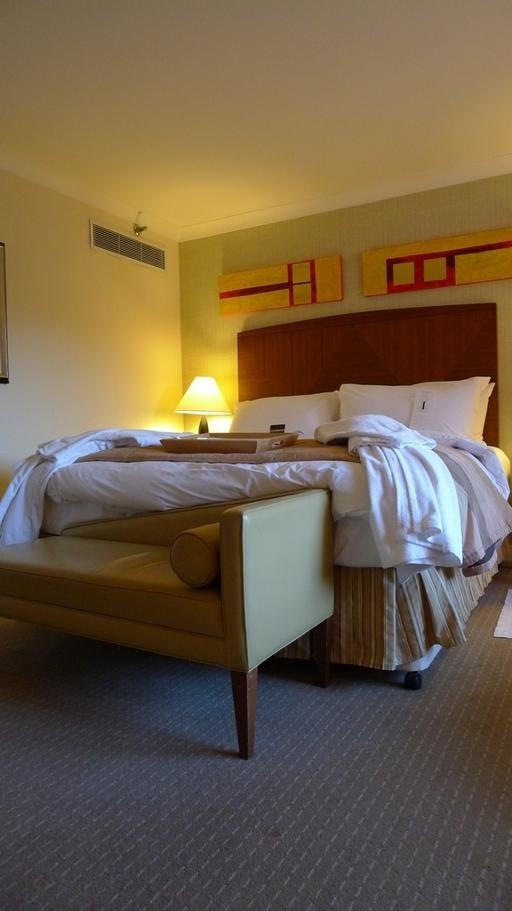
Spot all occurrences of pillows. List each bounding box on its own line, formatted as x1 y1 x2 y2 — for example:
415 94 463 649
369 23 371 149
230 375 497 445
168 523 222 589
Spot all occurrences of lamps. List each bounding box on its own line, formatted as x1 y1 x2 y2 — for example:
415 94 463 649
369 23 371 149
175 377 232 433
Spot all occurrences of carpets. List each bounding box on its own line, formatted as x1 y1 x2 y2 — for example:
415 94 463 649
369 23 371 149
494 584 511 639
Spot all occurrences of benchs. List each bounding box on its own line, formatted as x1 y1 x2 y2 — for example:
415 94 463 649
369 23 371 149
0 485 336 759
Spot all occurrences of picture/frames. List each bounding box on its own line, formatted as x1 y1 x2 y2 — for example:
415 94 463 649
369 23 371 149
0 242 10 383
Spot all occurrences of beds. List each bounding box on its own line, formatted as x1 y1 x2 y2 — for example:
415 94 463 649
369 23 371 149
0 301 511 689
